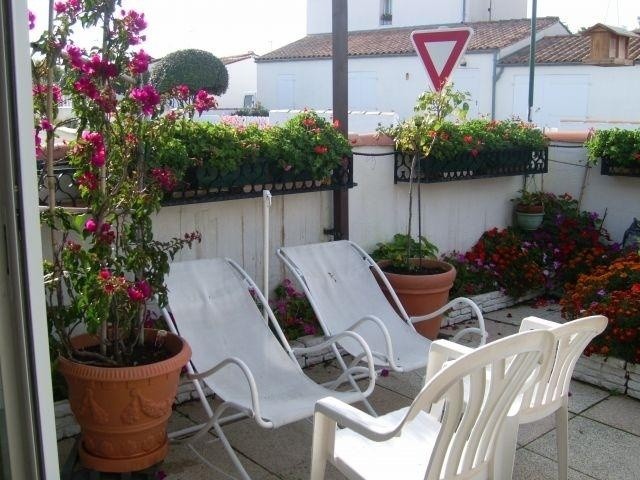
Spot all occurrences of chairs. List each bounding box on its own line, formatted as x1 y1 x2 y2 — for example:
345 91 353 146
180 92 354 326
144 257 377 480
274 239 489 418
309 326 558 480
422 314 610 480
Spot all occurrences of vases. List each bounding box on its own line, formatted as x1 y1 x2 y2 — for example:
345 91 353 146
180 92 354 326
58 328 193 473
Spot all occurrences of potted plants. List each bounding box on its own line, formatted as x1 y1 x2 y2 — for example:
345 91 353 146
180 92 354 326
511 189 545 230
369 80 472 342
583 125 639 178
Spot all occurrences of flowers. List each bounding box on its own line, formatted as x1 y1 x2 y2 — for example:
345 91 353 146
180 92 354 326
28 0 218 358
